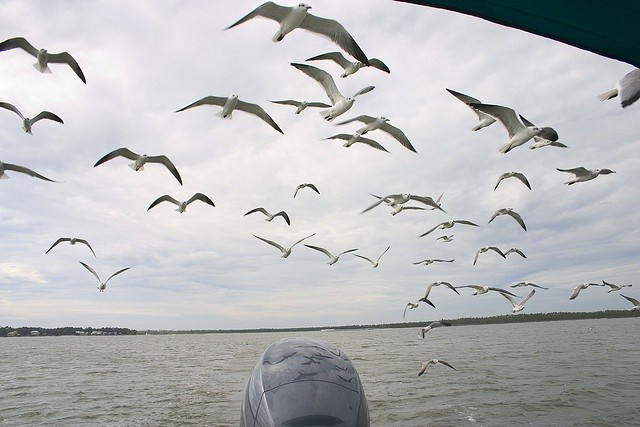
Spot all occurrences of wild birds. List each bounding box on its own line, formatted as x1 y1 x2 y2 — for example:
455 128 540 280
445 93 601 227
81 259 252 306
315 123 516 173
146 193 215 213
0 37 86 84
446 89 497 131
320 133 390 153
174 93 284 134
435 235 455 243
417 322 451 340
353 246 390 268
494 171 531 192
569 282 605 300
294 183 320 198
305 51 390 78
500 290 535 313
413 259 455 265
465 102 558 154
79 261 131 293
488 207 527 232
423 281 460 303
360 193 448 216
291 63 375 123
473 246 506 266
504 247 527 258
424 193 445 210
418 220 481 238
268 99 332 114
304 244 358 266
369 192 428 216
418 359 457 377
93 148 182 185
619 293 640 313
0 102 64 135
223 1 370 67
453 285 523 298
556 167 616 186
602 280 632 293
403 298 436 320
254 233 316 258
519 113 568 149
510 282 549 290
45 237 97 258
597 69 640 108
336 115 419 154
0 161 58 182
243 208 290 226
